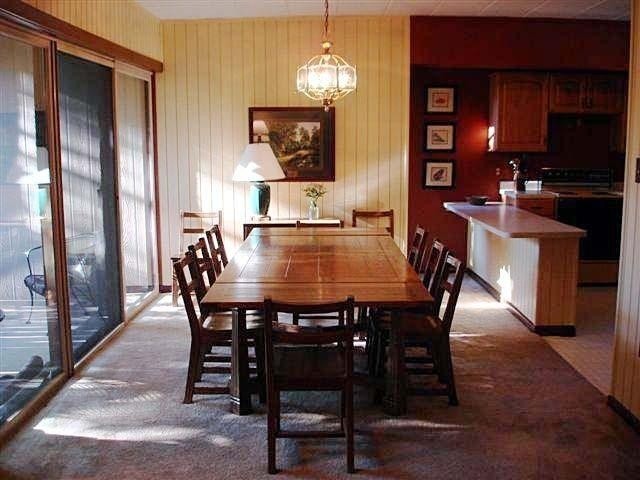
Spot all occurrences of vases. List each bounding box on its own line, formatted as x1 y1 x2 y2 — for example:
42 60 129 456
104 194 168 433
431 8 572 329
308 202 319 220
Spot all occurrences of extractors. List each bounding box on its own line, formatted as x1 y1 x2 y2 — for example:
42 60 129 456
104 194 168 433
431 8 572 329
548 112 621 129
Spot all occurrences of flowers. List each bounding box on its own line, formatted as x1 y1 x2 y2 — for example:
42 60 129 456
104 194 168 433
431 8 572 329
302 183 326 205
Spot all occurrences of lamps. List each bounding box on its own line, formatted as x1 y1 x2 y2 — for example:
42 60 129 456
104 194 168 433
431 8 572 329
297 1 356 113
233 143 286 220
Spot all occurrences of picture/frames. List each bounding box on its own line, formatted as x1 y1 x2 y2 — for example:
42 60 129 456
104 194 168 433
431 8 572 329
423 160 456 190
248 107 336 183
425 85 456 115
424 124 456 152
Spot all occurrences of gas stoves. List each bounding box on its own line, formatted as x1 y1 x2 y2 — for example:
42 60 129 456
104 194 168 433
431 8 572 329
542 185 624 199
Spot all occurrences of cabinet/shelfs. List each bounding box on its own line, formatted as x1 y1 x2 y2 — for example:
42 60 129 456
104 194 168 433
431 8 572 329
548 72 625 113
243 219 341 245
488 72 548 154
513 196 554 220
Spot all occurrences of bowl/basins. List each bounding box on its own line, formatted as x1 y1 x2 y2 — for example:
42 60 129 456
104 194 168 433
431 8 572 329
464 195 488 205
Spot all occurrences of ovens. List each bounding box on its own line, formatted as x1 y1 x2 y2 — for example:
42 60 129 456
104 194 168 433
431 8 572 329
557 199 623 259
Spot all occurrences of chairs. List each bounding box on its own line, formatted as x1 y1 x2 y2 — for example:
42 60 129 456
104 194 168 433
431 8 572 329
174 252 267 403
188 240 217 289
206 224 229 276
405 227 426 270
374 252 465 408
23 232 97 326
171 208 221 307
351 211 397 237
263 294 357 474
376 239 449 319
